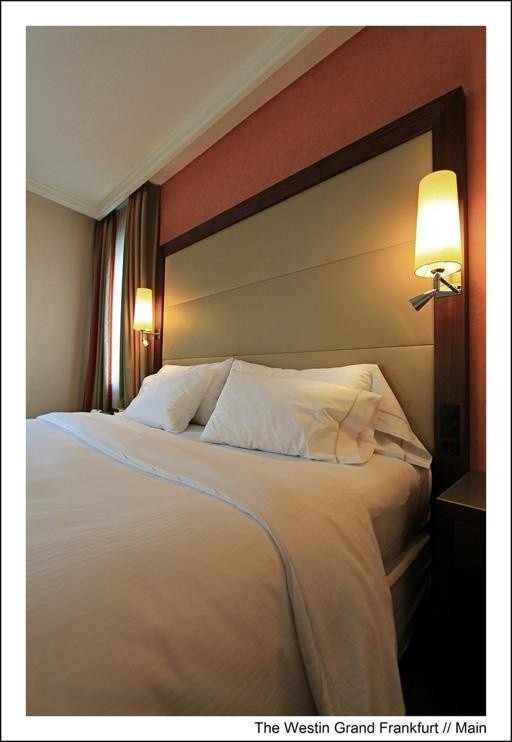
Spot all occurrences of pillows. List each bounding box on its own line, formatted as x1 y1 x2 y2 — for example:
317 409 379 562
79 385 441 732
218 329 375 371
193 356 234 427
275 363 433 469
122 364 214 434
199 358 382 465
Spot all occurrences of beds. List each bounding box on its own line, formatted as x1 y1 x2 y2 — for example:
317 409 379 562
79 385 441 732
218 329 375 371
25 85 475 714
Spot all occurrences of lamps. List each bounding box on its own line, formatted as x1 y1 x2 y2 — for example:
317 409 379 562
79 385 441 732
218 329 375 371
133 287 161 347
409 169 462 311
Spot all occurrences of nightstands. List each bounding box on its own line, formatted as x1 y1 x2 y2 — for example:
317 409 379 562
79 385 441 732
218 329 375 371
432 467 486 664
